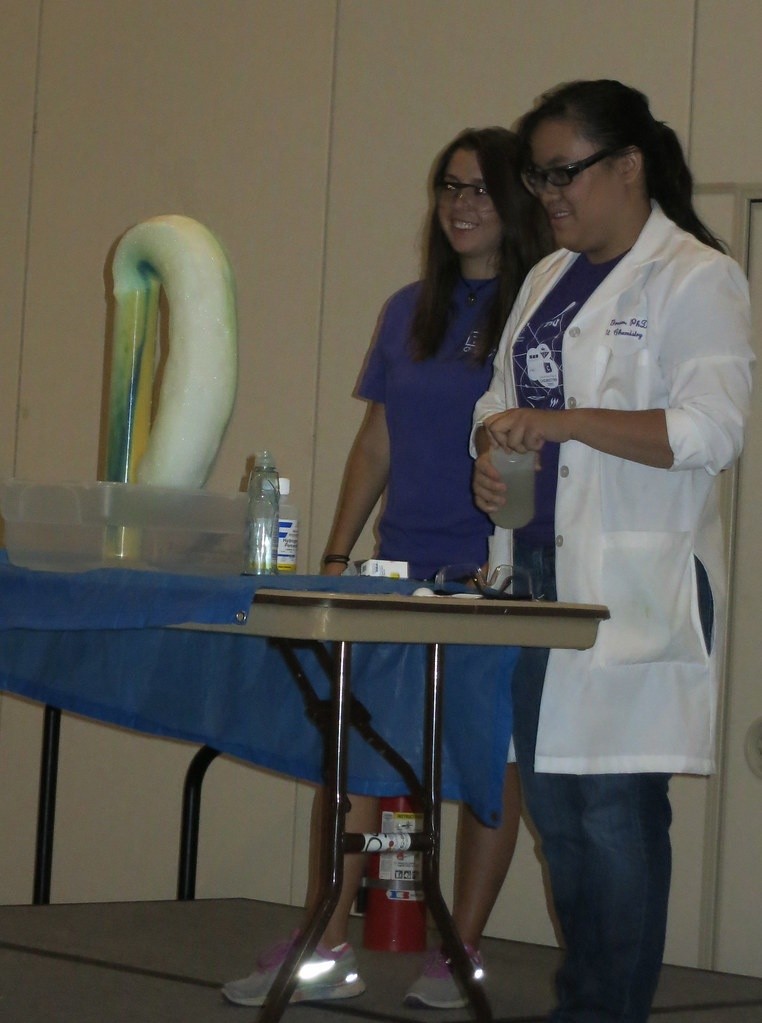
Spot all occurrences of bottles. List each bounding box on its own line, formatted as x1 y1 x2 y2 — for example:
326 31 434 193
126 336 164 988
488 443 536 529
264 477 298 573
246 450 279 576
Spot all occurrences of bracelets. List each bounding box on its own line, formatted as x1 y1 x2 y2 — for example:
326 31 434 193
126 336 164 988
324 555 350 565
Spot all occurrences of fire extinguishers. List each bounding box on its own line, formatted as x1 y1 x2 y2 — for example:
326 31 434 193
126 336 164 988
363 793 427 951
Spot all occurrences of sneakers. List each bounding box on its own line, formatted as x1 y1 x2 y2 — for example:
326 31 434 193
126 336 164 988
404 940 487 1007
221 928 366 1006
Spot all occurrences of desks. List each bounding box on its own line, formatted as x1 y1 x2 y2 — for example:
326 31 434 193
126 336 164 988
1 572 609 1023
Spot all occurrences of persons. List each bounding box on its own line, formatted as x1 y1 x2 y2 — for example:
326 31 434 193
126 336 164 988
218 130 561 1010
468 78 758 1023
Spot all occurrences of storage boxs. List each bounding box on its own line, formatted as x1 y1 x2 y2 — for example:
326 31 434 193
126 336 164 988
0 479 249 576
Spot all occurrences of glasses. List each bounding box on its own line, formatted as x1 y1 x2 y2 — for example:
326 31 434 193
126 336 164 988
434 179 498 212
433 562 535 602
521 148 612 197
